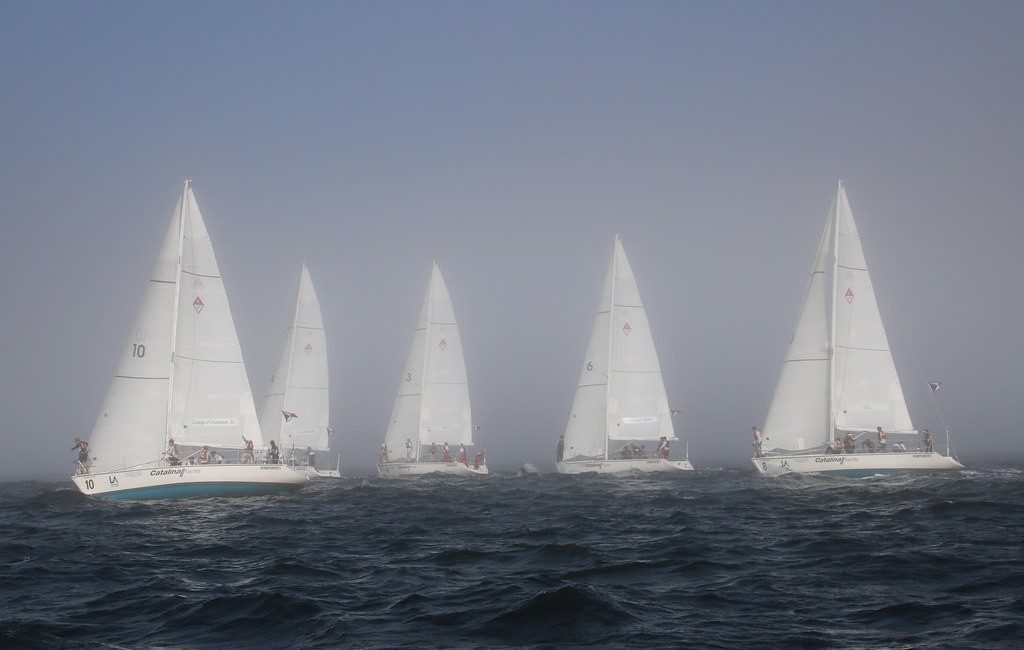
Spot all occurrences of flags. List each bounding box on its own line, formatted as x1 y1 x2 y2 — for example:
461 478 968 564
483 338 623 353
928 382 942 394
326 428 334 435
282 410 298 421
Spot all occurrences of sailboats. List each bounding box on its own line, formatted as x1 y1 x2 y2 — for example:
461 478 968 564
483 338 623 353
749 175 966 476
71 176 312 502
260 264 345 484
555 232 697 476
375 260 489 477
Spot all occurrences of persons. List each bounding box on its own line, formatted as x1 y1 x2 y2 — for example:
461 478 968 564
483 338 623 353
458 443 468 463
827 426 906 454
619 437 670 459
429 442 437 462
380 440 390 464
305 447 317 465
241 437 253 463
922 429 933 453
473 452 481 469
269 441 280 463
442 443 451 462
751 427 763 457
186 446 226 464
406 438 413 462
163 440 182 465
556 436 565 464
71 438 90 474
